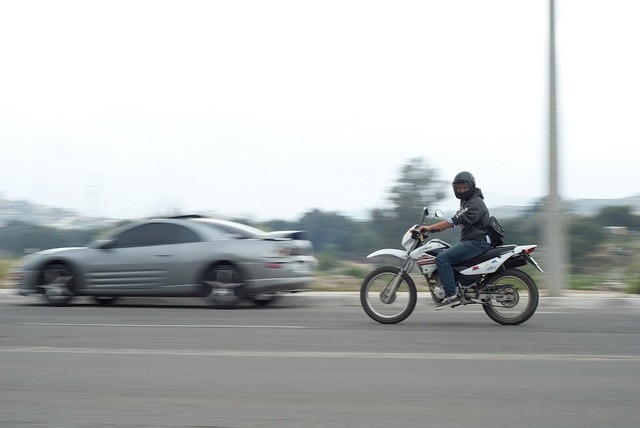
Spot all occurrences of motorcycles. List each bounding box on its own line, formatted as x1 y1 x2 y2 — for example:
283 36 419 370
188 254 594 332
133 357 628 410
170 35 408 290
360 207 545 324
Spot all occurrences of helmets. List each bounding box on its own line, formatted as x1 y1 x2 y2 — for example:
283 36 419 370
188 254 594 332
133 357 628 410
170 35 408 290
452 171 476 199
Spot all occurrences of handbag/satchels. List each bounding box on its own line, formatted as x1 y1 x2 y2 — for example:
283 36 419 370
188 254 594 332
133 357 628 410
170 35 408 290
484 216 504 247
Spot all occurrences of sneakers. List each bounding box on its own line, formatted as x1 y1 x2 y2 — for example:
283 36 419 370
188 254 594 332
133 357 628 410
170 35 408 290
435 296 461 310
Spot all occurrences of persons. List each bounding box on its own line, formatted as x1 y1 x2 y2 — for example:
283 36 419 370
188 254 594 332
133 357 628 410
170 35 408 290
417 170 492 311
